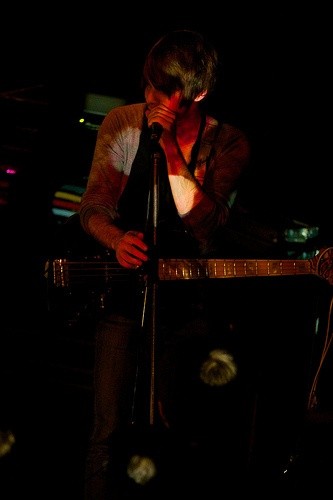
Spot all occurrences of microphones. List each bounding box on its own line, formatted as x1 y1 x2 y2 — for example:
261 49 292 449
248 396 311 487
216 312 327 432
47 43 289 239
150 122 163 140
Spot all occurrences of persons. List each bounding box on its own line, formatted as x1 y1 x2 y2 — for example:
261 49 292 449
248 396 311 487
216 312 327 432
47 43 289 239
78 27 249 500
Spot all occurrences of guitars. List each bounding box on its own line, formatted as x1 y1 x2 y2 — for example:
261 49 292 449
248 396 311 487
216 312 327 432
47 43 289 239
0 227 333 320
